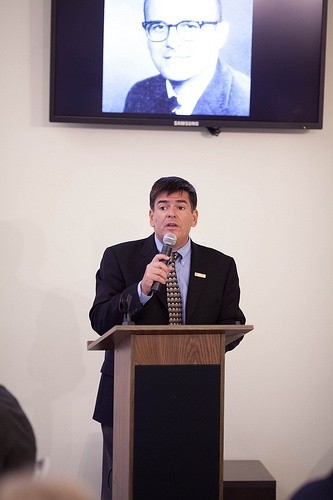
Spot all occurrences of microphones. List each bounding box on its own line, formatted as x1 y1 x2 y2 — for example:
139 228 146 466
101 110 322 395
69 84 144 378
149 232 178 293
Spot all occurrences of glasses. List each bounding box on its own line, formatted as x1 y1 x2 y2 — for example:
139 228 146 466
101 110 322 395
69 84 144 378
142 19 218 42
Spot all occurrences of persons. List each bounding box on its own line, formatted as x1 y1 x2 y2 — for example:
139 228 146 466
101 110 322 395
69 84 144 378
121 0 250 116
0 383 94 500
88 176 245 500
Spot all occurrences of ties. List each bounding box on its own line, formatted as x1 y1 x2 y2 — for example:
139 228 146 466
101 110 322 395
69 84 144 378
166 252 183 325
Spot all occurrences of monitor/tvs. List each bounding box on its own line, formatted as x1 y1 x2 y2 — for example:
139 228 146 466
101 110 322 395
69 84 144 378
48 0 328 130
